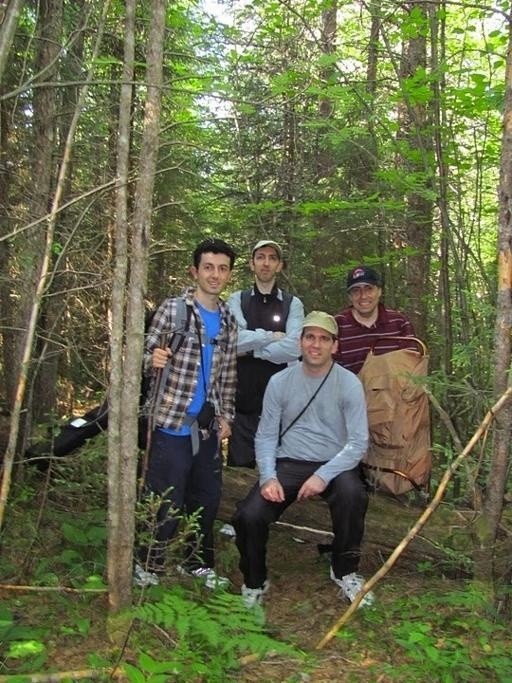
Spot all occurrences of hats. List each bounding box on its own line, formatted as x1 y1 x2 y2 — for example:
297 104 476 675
346 266 385 294
253 239 283 255
302 309 339 336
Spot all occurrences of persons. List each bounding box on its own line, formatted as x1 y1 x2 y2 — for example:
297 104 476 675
326 265 422 376
230 309 378 613
216 238 306 540
131 239 240 594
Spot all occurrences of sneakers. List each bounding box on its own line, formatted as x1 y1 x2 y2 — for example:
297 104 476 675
329 566 376 610
240 579 271 609
175 559 228 592
134 565 161 587
